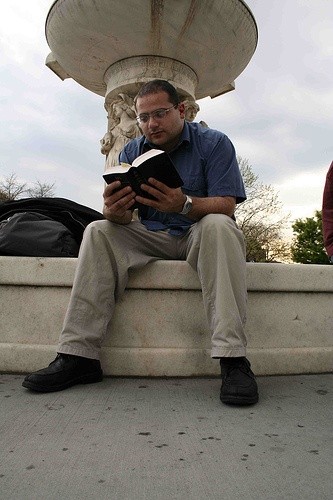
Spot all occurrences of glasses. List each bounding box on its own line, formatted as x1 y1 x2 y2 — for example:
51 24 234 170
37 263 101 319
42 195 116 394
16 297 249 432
136 105 177 123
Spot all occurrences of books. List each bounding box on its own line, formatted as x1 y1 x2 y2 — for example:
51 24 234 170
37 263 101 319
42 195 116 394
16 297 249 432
102 149 184 210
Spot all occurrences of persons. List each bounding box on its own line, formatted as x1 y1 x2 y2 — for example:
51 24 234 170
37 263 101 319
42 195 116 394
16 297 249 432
322 162 333 264
22 79 258 406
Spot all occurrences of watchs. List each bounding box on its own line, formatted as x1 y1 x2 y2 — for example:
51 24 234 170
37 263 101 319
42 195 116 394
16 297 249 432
178 195 193 215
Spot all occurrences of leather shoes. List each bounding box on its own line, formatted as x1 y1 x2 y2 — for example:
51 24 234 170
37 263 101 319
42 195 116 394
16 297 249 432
22 353 105 391
219 358 260 406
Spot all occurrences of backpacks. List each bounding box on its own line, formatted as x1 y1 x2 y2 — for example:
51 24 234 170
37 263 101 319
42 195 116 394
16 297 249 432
0 211 79 258
